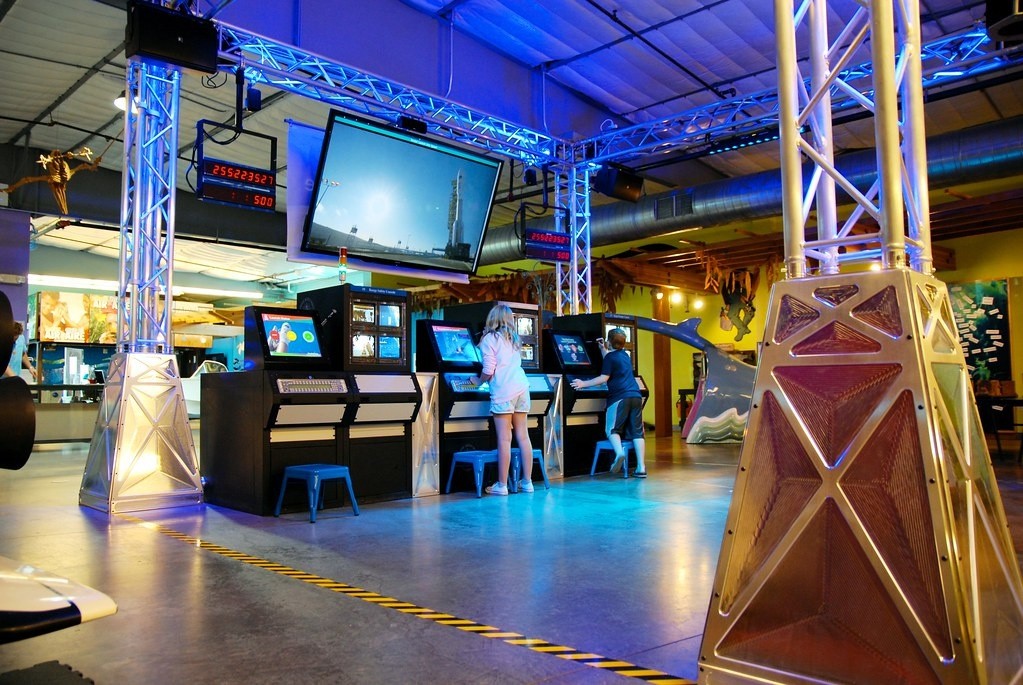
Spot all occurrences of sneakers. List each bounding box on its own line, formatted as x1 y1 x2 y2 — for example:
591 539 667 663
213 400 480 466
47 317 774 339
517 479 534 493
486 482 508 496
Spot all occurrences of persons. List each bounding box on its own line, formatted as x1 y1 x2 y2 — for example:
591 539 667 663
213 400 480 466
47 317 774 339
470 304 533 496
570 328 647 478
0 320 38 380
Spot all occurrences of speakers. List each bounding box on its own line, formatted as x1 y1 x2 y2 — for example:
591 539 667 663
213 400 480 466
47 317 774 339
246 88 262 112
125 6 218 74
397 116 428 134
595 168 643 203
524 169 537 186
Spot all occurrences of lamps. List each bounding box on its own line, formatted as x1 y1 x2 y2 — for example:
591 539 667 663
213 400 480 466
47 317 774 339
588 163 645 205
113 80 146 114
247 88 262 113
397 115 427 136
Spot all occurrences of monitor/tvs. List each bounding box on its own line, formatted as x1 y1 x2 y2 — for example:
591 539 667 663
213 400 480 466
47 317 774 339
261 313 321 357
300 108 505 276
432 325 479 362
554 334 592 365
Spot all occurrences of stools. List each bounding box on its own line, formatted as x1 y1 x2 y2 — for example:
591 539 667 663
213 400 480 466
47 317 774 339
274 464 360 523
445 448 550 498
590 439 634 479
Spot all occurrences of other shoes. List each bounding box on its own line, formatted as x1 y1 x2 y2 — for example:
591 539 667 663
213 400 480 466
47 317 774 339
632 469 647 478
610 451 626 475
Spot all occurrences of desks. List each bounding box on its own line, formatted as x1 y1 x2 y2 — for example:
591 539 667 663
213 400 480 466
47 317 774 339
974 395 1023 466
